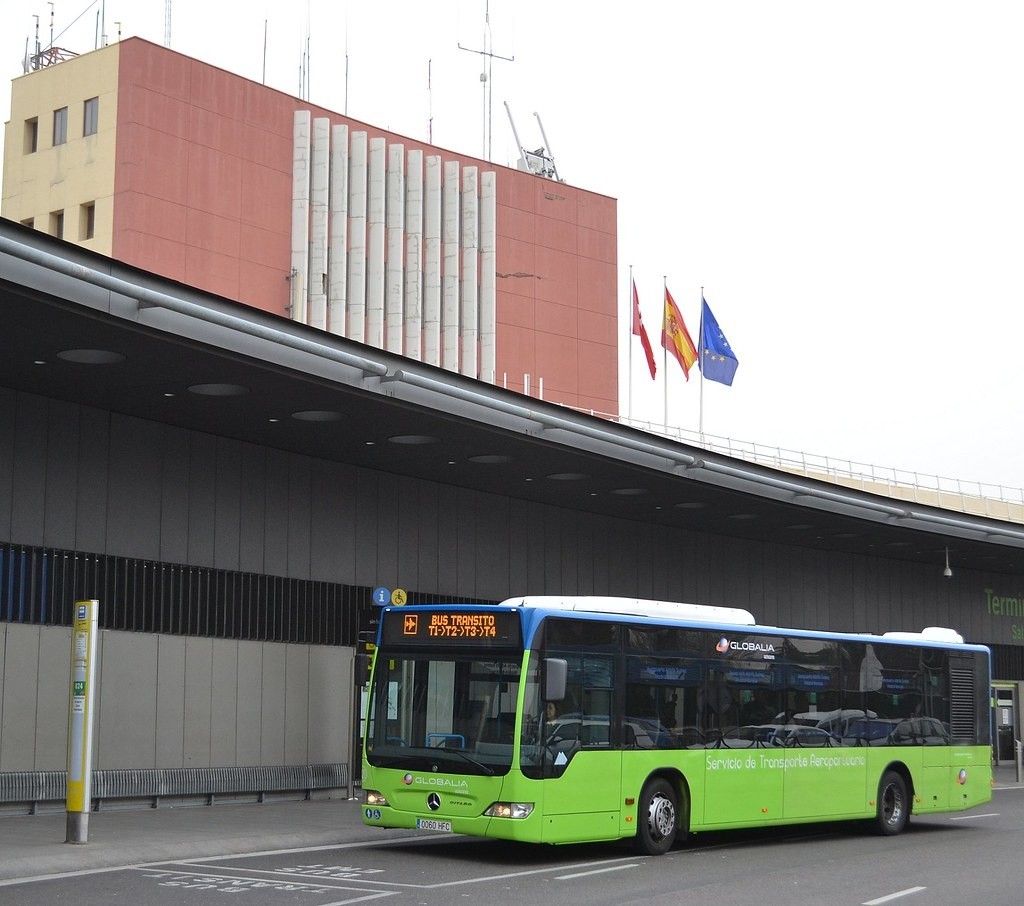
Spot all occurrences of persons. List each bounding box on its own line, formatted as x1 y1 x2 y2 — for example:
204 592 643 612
536 700 565 742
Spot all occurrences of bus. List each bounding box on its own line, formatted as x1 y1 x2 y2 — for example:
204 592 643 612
353 594 993 856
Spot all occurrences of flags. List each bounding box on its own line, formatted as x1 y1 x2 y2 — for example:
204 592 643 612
697 297 739 386
633 280 656 380
661 287 700 382
